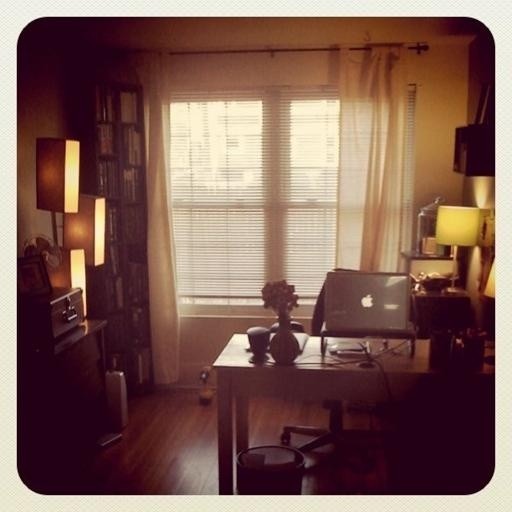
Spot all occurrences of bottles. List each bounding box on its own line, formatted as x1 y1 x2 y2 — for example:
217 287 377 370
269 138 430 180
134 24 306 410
415 195 447 257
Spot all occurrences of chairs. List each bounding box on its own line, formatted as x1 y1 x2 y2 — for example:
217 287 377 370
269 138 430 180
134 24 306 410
278 268 400 482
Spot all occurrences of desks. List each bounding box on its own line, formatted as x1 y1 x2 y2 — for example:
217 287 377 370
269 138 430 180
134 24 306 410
401 251 472 341
18 318 122 497
209 332 494 495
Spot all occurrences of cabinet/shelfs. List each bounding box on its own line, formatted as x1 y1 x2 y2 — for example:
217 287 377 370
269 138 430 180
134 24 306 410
83 58 155 399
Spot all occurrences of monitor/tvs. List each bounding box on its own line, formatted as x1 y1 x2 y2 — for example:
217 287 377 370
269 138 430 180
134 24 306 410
325 271 411 331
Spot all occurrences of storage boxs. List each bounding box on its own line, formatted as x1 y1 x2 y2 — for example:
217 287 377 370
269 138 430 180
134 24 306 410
20 257 86 337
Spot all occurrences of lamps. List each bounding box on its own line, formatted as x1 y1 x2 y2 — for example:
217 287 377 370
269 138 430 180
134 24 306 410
36 138 109 325
435 205 481 295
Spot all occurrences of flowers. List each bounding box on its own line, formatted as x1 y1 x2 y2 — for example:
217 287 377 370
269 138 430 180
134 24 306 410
262 280 299 320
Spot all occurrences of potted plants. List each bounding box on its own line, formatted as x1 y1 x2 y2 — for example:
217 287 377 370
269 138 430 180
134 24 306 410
268 315 301 369
